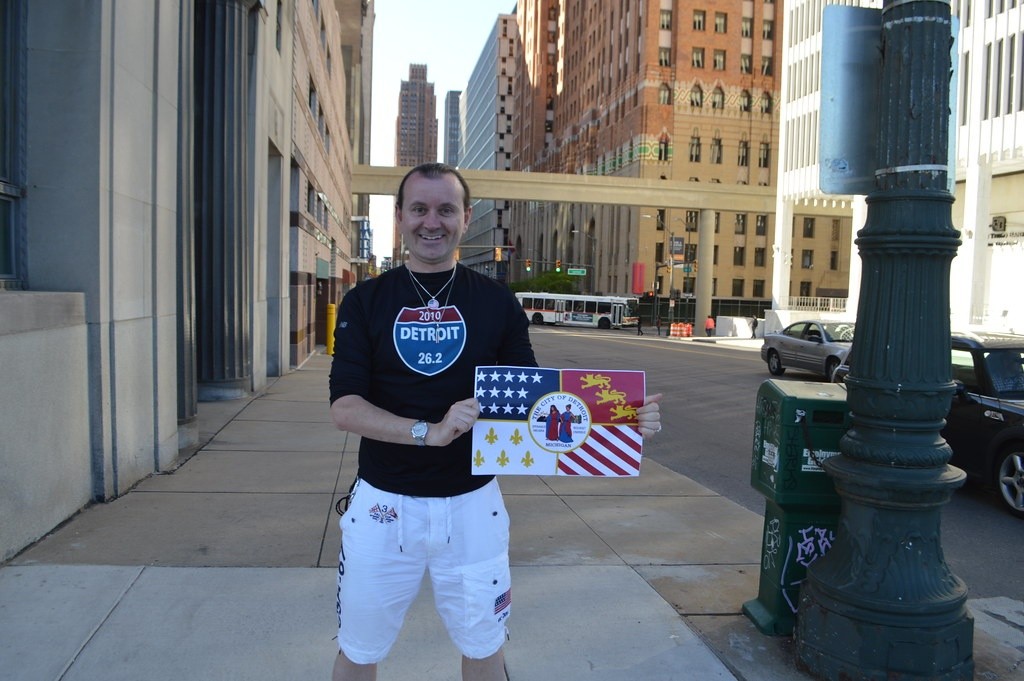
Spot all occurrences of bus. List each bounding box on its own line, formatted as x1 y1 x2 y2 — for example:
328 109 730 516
515 290 641 330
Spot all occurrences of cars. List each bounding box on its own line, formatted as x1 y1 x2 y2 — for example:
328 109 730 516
760 318 856 381
830 329 1024 520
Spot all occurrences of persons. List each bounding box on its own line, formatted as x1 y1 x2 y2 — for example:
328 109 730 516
328 163 663 681
637 315 643 335
705 315 714 336
747 313 757 339
656 315 662 337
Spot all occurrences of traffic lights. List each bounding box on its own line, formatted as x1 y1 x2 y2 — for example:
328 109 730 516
693 260 698 272
667 266 671 273
556 260 561 272
649 291 653 297
525 259 531 272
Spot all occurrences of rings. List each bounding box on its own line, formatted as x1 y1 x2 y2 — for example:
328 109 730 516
655 423 662 433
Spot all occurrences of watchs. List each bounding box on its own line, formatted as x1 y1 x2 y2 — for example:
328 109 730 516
412 417 428 448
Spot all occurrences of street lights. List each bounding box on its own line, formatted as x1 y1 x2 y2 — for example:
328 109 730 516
674 216 692 323
571 229 596 295
642 214 675 336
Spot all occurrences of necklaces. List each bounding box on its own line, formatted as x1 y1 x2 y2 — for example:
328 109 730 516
404 259 457 343
